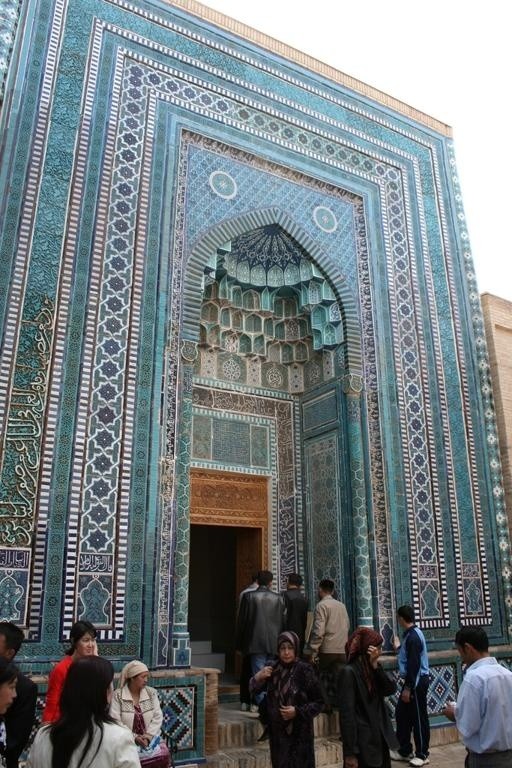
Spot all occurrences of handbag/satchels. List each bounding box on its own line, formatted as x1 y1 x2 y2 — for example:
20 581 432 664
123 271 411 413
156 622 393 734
258 694 269 725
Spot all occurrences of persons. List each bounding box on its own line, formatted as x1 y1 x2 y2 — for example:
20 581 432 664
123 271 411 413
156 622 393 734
248 630 327 768
25 654 143 768
389 604 431 767
0 663 20 768
0 621 40 767
42 619 100 726
440 624 511 768
108 659 173 768
233 570 351 717
336 626 398 768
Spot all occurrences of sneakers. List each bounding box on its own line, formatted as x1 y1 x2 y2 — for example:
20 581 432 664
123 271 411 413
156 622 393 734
409 757 429 767
389 750 413 761
